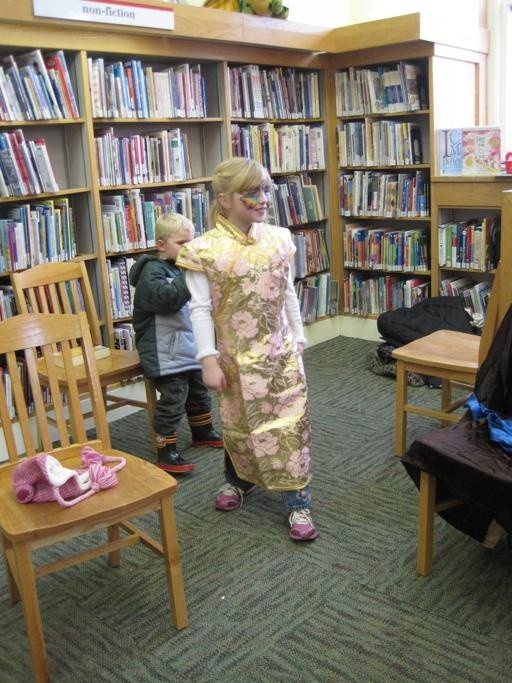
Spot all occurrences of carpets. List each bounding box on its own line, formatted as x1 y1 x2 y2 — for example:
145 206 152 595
0 336 510 683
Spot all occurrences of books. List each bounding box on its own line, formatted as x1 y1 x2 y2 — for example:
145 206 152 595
438 126 501 180
333 116 423 168
48 344 111 368
1 275 87 322
293 273 339 325
231 123 324 173
0 49 79 121
228 63 321 119
265 174 322 227
0 198 77 274
95 127 193 186
438 215 500 273
341 271 430 318
438 277 490 328
339 169 431 219
113 321 144 383
343 222 432 273
108 256 137 319
101 184 209 253
87 56 210 119
333 61 429 115
0 129 59 197
0 341 53 422
289 227 331 278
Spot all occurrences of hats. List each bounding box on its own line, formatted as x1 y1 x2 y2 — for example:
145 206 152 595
13 446 126 507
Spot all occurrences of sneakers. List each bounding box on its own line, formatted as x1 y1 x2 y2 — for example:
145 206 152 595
215 484 256 511
288 510 318 540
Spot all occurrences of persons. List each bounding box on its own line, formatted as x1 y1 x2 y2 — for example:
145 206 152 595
176 157 319 541
130 213 223 474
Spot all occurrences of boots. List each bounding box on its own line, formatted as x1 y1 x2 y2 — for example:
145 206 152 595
155 432 195 469
189 413 224 448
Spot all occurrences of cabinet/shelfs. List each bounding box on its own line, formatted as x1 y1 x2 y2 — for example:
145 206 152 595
435 268 497 332
0 26 478 427
434 176 512 323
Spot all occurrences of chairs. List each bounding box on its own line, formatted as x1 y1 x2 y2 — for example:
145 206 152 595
7 259 159 461
392 256 501 457
0 309 189 676
399 302 512 578
